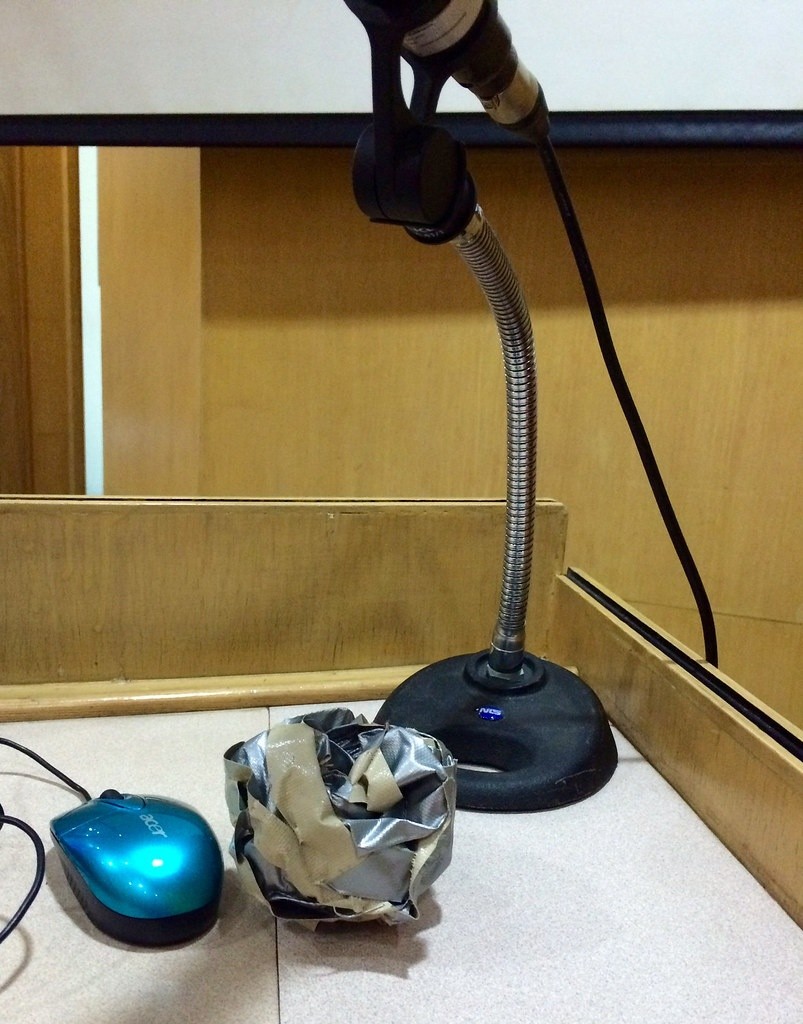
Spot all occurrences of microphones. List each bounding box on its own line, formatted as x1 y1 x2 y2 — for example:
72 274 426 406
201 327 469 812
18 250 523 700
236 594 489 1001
344 0 553 138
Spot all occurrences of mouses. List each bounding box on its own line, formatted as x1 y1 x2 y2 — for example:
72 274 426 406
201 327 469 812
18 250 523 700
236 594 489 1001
48 790 223 947
